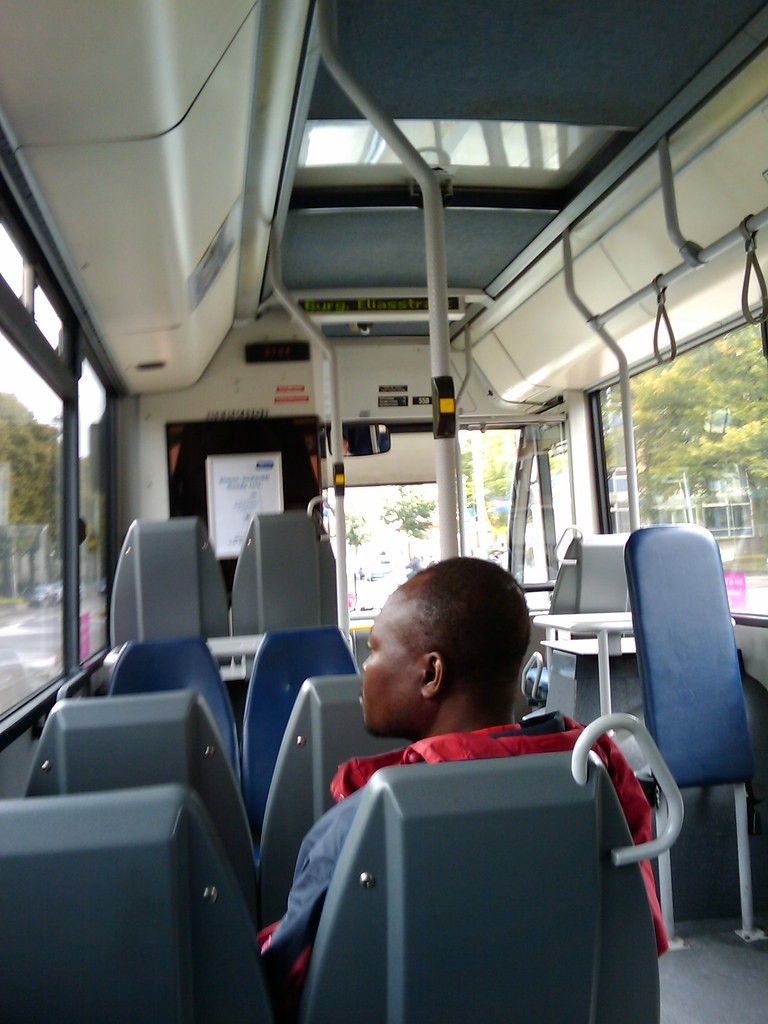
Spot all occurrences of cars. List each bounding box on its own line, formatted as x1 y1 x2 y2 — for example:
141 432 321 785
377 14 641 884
28 577 68 608
348 520 442 587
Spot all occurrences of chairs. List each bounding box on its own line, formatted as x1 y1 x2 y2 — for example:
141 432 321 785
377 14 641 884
522 528 632 711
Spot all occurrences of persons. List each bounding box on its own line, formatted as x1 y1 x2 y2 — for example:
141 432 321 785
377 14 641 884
342 427 352 456
255 557 669 1024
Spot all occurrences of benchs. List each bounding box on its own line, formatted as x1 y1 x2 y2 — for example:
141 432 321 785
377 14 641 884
0 509 684 1024
